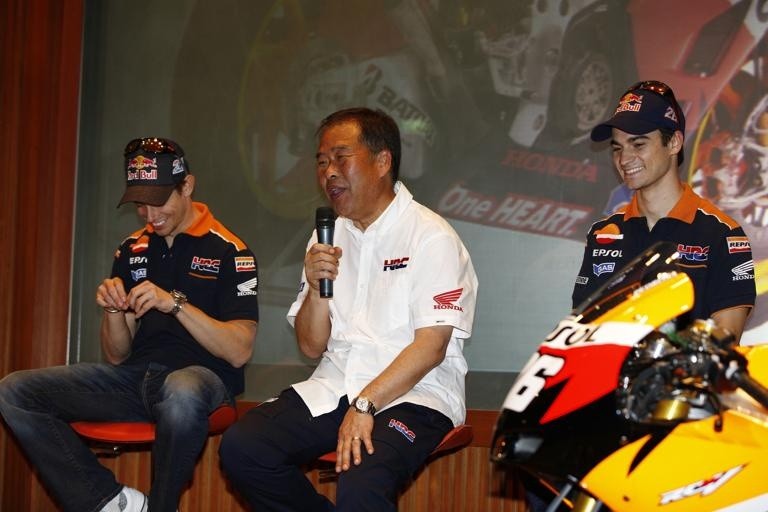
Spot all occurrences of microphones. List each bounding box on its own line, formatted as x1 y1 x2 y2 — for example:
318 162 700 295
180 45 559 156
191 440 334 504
315 206 335 299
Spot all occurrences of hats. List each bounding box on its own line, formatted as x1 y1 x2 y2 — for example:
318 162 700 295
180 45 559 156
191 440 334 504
114 136 190 210
590 90 687 144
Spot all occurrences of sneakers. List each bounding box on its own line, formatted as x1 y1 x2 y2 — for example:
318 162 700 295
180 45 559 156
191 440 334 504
97 486 149 511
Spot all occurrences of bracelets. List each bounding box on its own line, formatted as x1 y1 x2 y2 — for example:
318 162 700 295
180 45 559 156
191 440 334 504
102 305 122 314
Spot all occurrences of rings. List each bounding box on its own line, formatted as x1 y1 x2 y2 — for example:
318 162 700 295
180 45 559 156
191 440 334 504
349 435 362 442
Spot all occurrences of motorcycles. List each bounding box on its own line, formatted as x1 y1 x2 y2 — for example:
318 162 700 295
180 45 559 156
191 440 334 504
487 240 768 512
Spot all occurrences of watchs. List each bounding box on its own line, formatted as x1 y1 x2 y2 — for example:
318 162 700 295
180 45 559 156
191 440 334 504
164 288 189 317
348 397 378 419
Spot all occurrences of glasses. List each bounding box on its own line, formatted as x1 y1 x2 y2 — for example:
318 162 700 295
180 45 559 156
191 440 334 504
621 79 685 98
124 138 189 174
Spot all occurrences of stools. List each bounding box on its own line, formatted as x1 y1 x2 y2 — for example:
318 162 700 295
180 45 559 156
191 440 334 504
70 405 237 498
318 425 472 463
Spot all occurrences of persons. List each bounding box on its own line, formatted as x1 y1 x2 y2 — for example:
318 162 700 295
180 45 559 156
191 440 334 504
567 78 759 352
215 105 479 509
1 137 260 512
